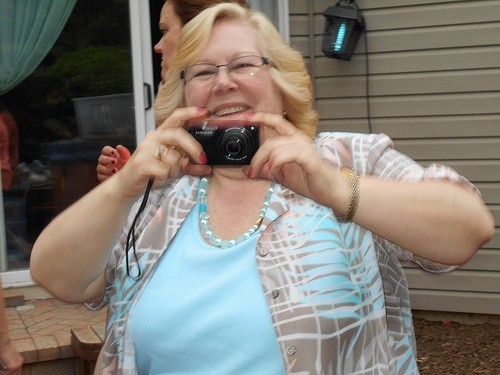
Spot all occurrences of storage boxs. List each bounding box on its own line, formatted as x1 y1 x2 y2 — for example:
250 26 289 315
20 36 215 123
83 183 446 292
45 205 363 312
72 93 136 139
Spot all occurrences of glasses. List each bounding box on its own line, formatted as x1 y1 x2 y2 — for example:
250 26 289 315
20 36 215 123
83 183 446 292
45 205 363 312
180 54 276 88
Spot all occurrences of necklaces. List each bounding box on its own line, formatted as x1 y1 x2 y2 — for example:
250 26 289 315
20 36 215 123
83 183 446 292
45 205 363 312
199 175 275 248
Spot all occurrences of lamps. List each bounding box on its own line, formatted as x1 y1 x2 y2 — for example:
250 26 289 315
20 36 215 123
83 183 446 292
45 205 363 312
319 0 366 62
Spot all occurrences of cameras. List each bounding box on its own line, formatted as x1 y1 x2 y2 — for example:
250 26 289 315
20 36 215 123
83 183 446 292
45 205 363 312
190 124 259 165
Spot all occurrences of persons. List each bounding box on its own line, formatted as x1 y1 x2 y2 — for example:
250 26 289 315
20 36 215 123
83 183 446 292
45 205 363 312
0 111 32 260
30 3 495 375
96 0 251 181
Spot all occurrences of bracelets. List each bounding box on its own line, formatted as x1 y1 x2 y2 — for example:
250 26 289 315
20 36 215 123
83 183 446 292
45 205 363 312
333 166 360 222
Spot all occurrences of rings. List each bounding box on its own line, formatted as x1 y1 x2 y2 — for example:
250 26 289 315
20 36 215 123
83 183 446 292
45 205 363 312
158 144 170 163
